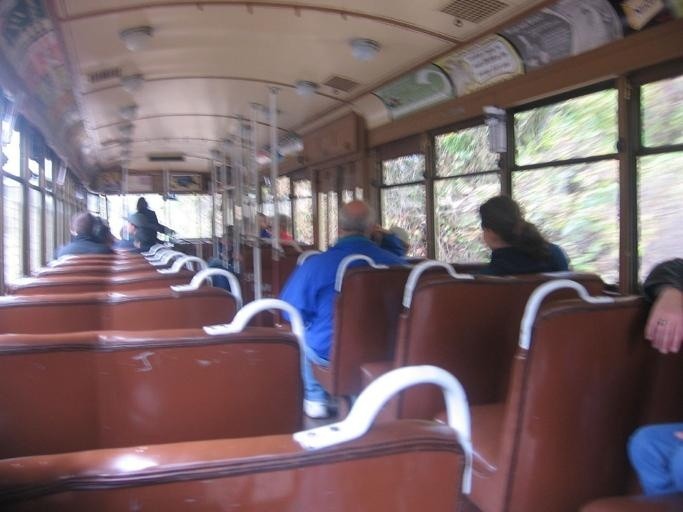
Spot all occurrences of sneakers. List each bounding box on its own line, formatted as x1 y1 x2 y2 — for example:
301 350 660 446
302 398 330 418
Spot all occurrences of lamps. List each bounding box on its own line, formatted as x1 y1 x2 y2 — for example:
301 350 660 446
116 26 381 160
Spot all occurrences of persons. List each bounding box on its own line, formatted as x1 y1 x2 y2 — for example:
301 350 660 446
55 211 117 254
74 212 164 254
621 258 683 500
135 197 176 244
218 211 291 269
474 195 573 276
375 222 414 260
276 198 409 421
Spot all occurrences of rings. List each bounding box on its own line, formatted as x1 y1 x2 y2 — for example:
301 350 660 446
656 319 666 327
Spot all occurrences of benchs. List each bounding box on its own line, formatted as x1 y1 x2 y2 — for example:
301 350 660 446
2 235 682 512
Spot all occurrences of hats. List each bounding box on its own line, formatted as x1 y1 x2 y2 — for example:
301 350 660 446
121 212 150 228
